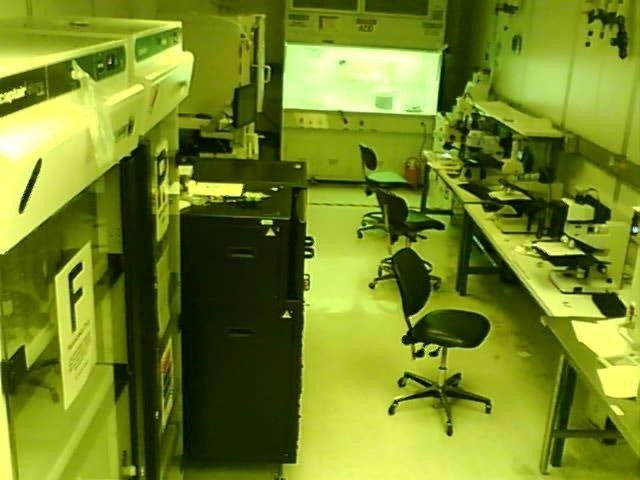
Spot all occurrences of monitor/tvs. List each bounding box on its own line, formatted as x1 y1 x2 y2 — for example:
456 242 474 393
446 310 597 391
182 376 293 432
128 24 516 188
232 83 257 129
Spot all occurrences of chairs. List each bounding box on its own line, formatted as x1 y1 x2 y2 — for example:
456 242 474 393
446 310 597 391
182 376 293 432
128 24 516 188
356 144 493 436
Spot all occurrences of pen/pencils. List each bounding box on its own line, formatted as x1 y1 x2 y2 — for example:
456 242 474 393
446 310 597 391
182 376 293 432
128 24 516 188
226 197 264 204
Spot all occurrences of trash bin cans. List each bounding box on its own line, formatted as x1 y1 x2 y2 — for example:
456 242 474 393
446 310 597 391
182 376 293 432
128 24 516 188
405 158 421 190
